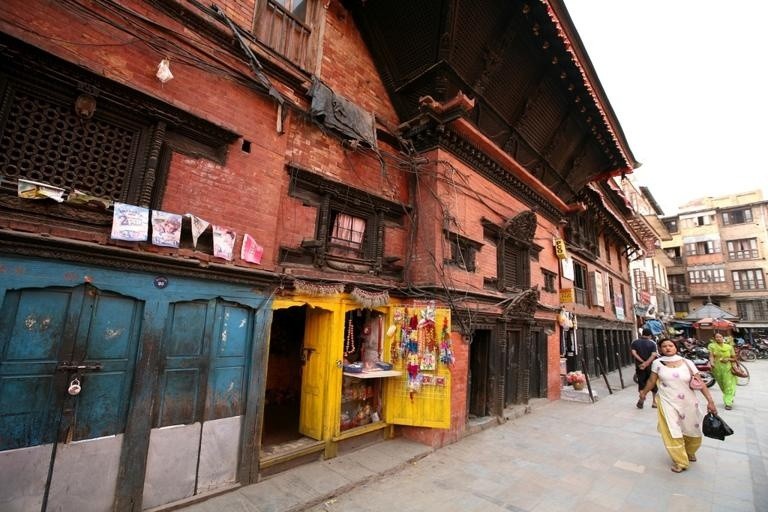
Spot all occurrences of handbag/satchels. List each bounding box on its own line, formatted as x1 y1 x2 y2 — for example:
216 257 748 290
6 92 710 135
731 366 748 378
689 376 704 390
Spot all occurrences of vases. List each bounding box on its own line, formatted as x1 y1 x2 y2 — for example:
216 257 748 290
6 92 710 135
573 382 584 391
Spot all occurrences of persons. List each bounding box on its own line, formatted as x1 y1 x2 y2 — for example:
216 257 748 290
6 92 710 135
632 328 658 408
707 332 737 409
639 338 717 473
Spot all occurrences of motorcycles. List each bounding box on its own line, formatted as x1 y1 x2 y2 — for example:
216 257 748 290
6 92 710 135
677 336 768 389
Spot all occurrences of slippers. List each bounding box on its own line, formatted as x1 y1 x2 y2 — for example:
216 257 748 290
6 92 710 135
671 465 683 472
688 453 696 461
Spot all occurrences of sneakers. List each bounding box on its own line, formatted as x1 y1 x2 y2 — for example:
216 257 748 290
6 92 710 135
636 399 644 408
725 405 731 410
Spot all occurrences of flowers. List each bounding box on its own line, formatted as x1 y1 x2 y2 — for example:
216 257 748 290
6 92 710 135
565 370 587 386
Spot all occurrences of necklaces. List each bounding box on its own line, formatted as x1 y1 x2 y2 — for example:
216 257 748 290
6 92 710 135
673 364 677 368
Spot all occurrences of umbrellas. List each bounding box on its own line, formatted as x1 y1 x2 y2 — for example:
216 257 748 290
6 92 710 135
691 317 736 335
